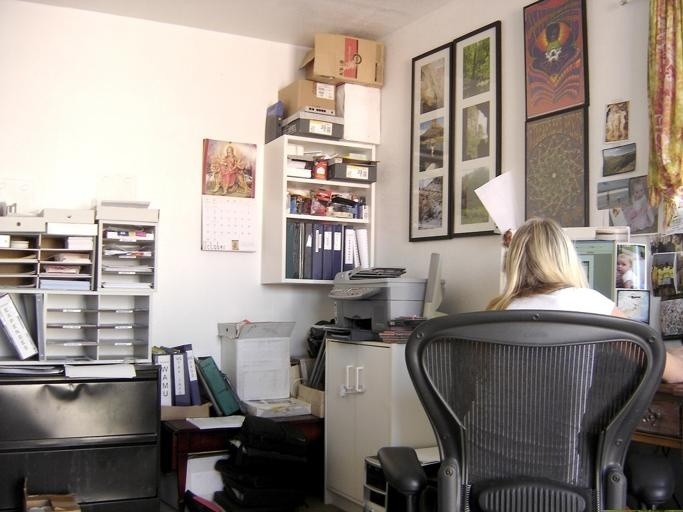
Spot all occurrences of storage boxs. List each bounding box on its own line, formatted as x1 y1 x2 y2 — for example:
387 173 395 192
294 32 387 89
296 383 324 419
22 476 80 512
336 81 381 149
326 155 381 184
216 319 296 403
275 78 338 120
279 110 344 141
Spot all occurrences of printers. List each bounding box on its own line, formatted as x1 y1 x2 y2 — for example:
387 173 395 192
312 252 445 341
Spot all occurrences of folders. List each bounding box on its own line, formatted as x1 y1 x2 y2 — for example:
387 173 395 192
171 344 202 406
303 223 355 280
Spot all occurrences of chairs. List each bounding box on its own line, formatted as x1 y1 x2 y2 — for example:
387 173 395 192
378 308 677 511
183 412 324 511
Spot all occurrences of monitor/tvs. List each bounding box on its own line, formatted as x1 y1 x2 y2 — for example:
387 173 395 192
499 239 618 306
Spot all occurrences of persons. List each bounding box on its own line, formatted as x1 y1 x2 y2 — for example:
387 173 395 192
623 180 655 233
463 219 683 487
650 234 683 296
617 253 640 288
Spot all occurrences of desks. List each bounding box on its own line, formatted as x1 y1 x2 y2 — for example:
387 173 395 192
162 396 326 512
629 378 682 452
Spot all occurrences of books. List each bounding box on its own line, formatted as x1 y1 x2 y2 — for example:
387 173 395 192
243 397 312 418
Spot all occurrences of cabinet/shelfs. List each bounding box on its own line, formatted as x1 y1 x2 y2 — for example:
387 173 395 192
261 135 376 289
0 364 164 512
0 198 163 365
320 334 440 512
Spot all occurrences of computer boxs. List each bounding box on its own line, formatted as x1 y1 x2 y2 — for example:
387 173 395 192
364 444 444 512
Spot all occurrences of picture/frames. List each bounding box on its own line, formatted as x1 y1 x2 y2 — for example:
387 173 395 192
521 0 592 121
406 40 454 243
447 17 503 241
521 103 592 231
